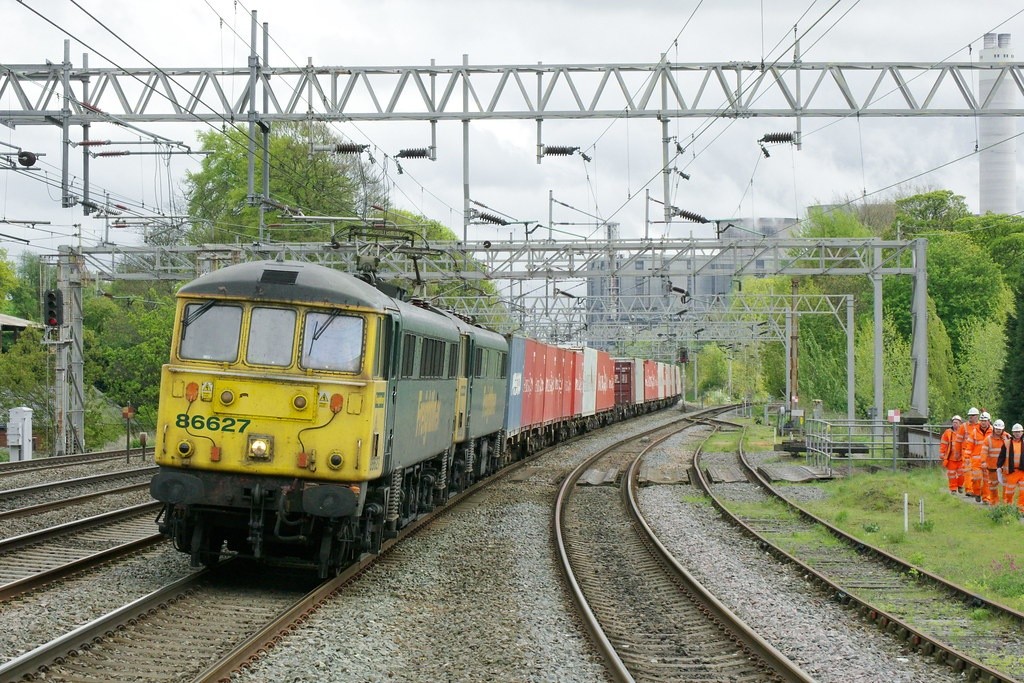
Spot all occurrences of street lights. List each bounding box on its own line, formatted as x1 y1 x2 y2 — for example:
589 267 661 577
672 308 688 409
693 328 704 401
372 205 431 299
717 321 768 399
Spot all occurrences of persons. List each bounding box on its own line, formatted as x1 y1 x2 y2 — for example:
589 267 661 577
940 407 1024 513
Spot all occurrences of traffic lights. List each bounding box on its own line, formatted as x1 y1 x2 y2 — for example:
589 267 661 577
678 346 689 363
44 290 64 326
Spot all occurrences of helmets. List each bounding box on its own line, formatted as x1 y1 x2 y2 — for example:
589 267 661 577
1012 424 1023 432
968 407 979 415
992 420 1005 430
952 414 963 422
980 411 991 422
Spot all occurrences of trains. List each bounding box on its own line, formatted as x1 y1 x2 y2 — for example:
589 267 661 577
148 256 683 590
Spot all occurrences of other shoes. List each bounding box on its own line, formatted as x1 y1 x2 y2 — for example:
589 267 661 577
975 495 981 502
983 501 989 505
966 493 972 496
957 486 964 493
952 490 957 495
